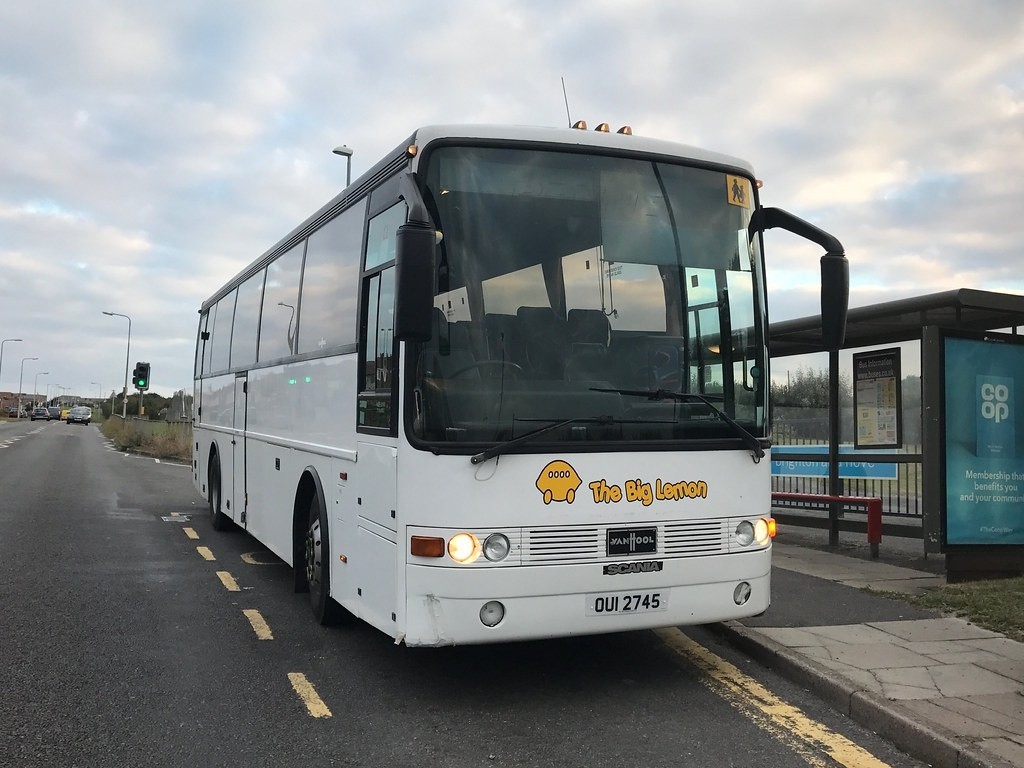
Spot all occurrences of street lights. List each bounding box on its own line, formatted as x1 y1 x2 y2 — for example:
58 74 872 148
0 339 23 373
103 312 131 418
46 383 71 407
333 145 353 188
91 382 101 417
18 358 38 418
34 372 48 408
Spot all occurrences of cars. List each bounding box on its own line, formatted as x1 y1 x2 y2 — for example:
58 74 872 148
8 408 28 418
31 406 93 426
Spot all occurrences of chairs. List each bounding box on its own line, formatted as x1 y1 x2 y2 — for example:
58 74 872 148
413 307 614 422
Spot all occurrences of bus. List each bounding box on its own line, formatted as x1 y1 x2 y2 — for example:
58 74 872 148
192 121 850 649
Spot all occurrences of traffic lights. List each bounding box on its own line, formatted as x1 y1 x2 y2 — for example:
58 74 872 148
132 363 149 390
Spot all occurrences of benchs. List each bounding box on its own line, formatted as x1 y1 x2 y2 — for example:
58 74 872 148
771 491 883 559
